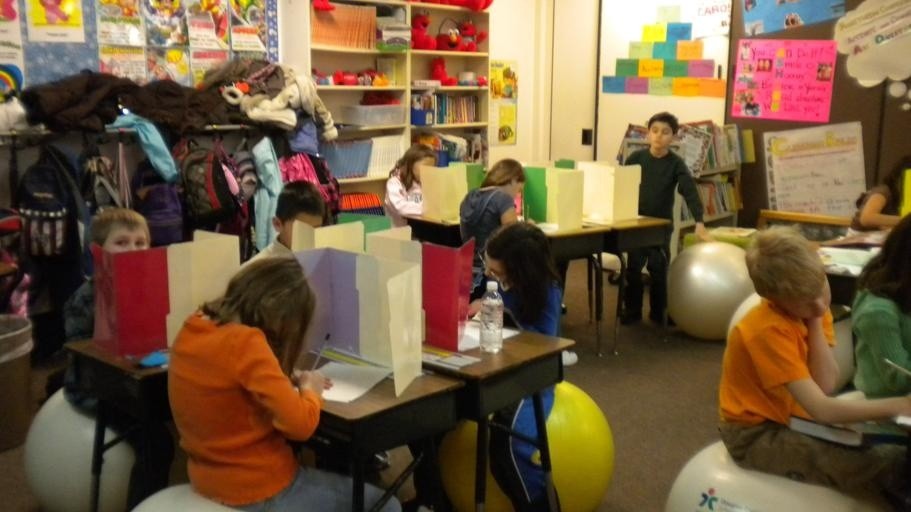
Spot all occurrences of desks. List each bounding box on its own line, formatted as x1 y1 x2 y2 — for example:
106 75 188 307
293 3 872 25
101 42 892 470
63 343 168 512
592 205 670 355
284 375 462 511
543 212 610 358
420 327 575 512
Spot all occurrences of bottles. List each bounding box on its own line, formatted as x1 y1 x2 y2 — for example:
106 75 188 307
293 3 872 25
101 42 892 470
478 280 503 354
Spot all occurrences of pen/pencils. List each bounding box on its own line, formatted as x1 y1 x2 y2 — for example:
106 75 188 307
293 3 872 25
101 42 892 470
311 331 332 371
883 357 911 378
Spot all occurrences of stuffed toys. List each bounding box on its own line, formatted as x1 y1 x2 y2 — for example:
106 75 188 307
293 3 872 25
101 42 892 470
464 20 489 50
412 13 433 48
436 27 462 51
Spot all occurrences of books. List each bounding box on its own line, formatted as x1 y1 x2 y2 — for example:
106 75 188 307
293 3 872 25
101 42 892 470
310 3 378 48
416 92 482 124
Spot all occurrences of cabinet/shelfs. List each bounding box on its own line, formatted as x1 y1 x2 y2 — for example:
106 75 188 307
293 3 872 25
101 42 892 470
1 111 304 230
299 0 491 181
672 156 739 254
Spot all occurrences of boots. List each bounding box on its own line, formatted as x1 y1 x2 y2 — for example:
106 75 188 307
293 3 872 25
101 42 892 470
648 285 677 328
620 268 644 325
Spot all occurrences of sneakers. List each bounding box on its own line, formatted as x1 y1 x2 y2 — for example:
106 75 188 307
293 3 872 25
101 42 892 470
562 349 580 367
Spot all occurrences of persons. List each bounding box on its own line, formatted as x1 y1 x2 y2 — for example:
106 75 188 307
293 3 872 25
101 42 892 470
464 221 568 511
167 256 403 511
383 143 435 229
846 159 907 240
620 111 710 326
456 158 526 302
237 179 326 272
847 215 905 420
62 209 181 512
718 224 907 507
